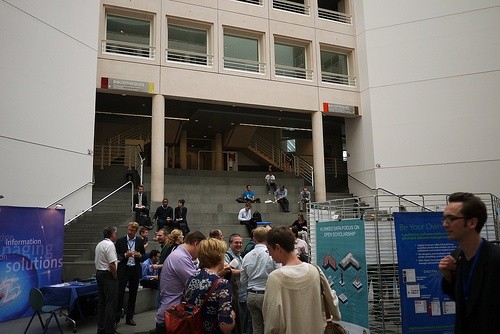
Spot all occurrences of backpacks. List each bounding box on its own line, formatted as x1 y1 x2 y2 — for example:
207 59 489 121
163 279 224 334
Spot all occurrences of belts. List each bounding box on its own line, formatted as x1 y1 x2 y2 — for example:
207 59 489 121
248 289 265 294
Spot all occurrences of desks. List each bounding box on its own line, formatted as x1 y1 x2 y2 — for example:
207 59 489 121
40 280 100 321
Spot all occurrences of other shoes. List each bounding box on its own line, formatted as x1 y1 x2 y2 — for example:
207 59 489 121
126 320 137 326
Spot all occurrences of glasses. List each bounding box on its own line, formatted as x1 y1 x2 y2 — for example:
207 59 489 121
441 216 465 224
234 241 242 244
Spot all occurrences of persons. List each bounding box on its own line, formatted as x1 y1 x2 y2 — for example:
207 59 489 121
299 185 311 213
291 213 308 242
352 196 365 220
94 224 120 333
262 225 341 334
136 229 149 247
242 184 261 203
274 185 290 213
237 202 257 235
242 230 255 257
239 227 284 334
153 197 175 231
153 228 170 248
180 237 236 334
114 221 146 327
439 193 500 334
288 226 310 261
223 233 243 298
154 229 206 333
174 199 191 237
264 171 277 195
141 248 163 288
125 165 140 189
209 228 223 240
130 184 151 226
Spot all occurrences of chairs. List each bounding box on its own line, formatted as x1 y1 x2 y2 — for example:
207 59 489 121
24 288 64 334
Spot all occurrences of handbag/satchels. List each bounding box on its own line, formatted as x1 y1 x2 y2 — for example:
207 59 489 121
324 321 346 334
